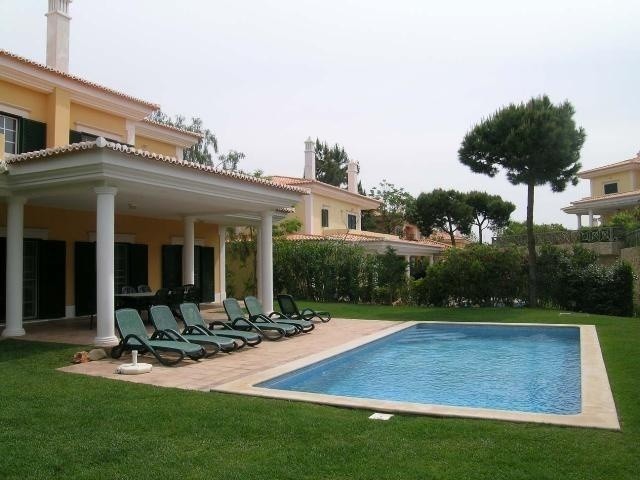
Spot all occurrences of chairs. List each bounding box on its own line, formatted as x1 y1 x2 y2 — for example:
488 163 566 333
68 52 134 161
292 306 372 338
89 284 331 366
